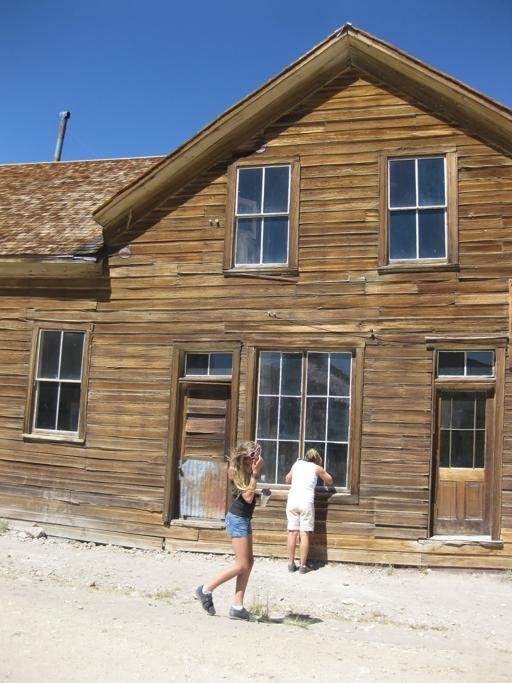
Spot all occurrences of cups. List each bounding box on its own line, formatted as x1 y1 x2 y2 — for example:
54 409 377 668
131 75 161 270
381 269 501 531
259 488 272 508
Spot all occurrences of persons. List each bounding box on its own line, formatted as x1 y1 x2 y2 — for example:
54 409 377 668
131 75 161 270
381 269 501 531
193 440 265 621
284 448 333 574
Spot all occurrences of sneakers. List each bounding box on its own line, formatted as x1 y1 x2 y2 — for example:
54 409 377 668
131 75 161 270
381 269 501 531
229 605 259 621
288 562 313 573
195 584 216 613
